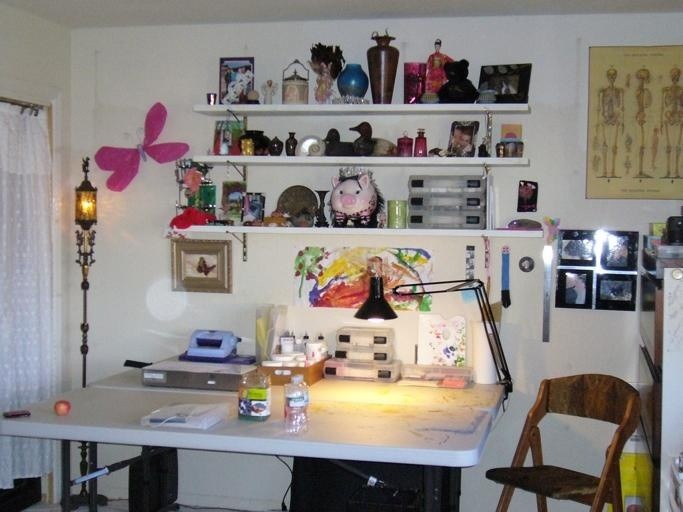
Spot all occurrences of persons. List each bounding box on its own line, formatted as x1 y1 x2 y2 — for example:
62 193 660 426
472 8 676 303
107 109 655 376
196 257 208 276
451 126 473 152
221 65 255 103
425 37 453 93
260 79 280 104
222 128 231 144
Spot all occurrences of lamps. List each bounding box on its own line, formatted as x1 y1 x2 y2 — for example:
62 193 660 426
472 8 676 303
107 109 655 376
76 157 97 388
354 256 514 394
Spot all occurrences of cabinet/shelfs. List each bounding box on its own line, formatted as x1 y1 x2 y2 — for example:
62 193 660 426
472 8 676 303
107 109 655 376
166 103 545 238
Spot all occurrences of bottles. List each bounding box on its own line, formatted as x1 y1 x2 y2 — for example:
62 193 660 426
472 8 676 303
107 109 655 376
315 334 326 354
297 375 309 424
338 63 368 99
268 136 283 156
283 377 304 434
414 128 426 157
285 132 297 156
237 129 271 156
396 130 413 157
301 333 310 356
368 31 399 104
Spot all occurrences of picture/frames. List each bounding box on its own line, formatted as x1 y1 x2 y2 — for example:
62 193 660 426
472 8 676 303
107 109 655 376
595 271 638 310
599 229 639 271
171 237 233 293
217 55 254 106
557 228 598 269
555 268 593 310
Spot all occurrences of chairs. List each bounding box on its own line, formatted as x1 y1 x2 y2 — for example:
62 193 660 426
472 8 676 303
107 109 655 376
485 372 641 512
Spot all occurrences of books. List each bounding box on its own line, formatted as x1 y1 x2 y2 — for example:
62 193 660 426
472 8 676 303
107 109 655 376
140 400 228 430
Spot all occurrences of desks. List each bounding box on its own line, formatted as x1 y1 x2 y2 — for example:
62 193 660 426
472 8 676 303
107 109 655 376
0 356 505 512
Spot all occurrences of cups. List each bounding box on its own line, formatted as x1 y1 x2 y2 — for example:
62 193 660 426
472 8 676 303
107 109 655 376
238 373 272 421
387 200 407 230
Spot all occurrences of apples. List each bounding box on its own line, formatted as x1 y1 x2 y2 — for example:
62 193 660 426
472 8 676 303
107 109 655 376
54 400 71 416
